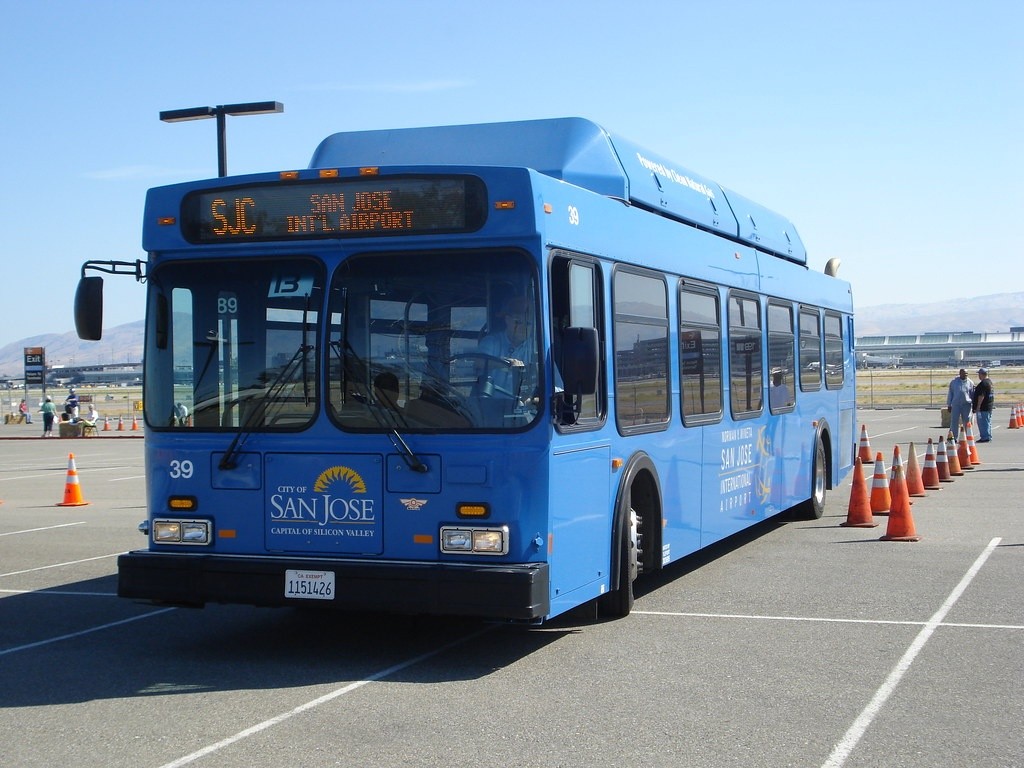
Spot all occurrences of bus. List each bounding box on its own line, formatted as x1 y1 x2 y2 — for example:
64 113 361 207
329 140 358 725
73 116 856 629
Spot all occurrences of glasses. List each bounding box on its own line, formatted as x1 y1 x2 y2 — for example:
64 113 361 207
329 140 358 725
514 320 532 325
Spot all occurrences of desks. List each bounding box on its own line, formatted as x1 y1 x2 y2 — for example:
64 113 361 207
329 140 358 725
59 420 84 437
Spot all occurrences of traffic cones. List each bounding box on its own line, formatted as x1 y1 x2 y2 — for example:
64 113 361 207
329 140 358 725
115 415 126 431
958 426 975 468
101 414 111 431
55 453 91 507
1020 403 1024 425
921 438 944 489
869 451 891 514
858 424 875 464
935 435 955 482
906 442 929 497
946 428 964 477
889 445 913 506
966 422 981 464
879 466 922 541
129 414 140 431
1016 404 1024 427
839 457 880 528
1006 406 1019 429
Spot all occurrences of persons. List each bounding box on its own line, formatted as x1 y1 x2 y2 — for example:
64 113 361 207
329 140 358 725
471 300 564 409
174 402 188 427
69 404 98 438
972 368 996 443
37 395 58 438
364 370 403 423
946 368 975 443
758 367 791 410
64 388 79 419
61 405 75 421
19 398 33 424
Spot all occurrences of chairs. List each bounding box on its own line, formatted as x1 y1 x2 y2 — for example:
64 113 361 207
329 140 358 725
82 419 99 437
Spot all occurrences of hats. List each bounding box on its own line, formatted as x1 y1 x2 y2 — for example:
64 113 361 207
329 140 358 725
47 396 50 399
771 367 782 374
496 298 533 318
976 368 988 373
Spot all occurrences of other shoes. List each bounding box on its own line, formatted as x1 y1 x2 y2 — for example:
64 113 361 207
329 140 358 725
976 439 990 443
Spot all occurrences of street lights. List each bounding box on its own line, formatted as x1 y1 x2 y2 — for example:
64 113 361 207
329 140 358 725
159 101 285 177
